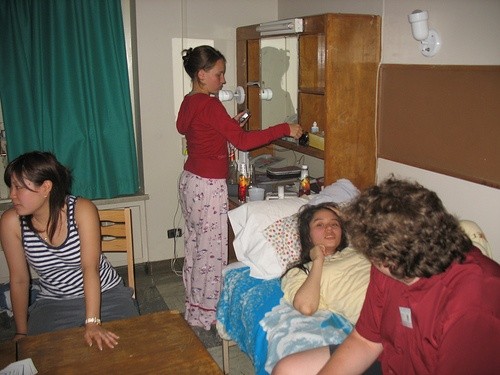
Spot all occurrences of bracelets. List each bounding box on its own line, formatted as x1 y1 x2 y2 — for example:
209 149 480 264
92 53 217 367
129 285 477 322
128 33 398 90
15 332 27 335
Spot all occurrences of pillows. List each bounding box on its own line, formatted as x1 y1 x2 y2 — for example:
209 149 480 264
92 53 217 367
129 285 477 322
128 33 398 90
227 178 363 280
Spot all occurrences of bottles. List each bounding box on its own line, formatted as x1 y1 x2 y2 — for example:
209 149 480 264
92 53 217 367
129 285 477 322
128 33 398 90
310 121 320 133
277 185 284 200
228 149 248 204
299 165 310 197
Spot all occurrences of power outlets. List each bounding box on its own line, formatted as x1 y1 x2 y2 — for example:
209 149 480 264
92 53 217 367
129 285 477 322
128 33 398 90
168 228 182 238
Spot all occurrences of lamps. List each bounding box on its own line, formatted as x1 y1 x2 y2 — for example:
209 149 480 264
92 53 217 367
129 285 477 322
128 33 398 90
260 89 273 100
256 19 303 36
408 9 441 58
219 86 245 105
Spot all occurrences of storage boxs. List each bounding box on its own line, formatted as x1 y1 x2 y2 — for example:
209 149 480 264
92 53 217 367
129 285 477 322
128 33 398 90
309 131 325 151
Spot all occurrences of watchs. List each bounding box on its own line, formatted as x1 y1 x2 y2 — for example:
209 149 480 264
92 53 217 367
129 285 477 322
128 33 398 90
85 317 101 326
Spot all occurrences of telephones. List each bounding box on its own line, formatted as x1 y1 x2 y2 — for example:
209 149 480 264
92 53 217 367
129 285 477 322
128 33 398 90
250 154 288 173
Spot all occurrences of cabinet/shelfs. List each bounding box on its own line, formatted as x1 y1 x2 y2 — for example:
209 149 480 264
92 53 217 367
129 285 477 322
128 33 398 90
236 12 382 193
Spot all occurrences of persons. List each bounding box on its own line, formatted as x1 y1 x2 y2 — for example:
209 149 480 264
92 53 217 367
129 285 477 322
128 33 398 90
280 201 370 328
175 46 304 331
270 174 500 375
0 151 139 350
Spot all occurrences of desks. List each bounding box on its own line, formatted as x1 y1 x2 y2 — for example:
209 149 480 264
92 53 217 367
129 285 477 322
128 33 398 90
17 310 226 375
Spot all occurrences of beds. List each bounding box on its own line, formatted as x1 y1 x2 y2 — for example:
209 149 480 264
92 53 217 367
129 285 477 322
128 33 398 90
215 261 354 375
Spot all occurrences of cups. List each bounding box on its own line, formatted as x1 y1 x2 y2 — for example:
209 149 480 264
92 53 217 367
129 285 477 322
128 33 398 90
249 188 265 201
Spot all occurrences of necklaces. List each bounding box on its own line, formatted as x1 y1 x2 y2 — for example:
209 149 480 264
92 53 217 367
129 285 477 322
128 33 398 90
39 231 48 241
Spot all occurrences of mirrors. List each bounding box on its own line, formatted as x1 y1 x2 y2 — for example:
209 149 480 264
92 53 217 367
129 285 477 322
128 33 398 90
246 37 299 143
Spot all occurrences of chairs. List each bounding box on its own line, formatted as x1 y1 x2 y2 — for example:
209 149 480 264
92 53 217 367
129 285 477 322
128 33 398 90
98 207 140 314
